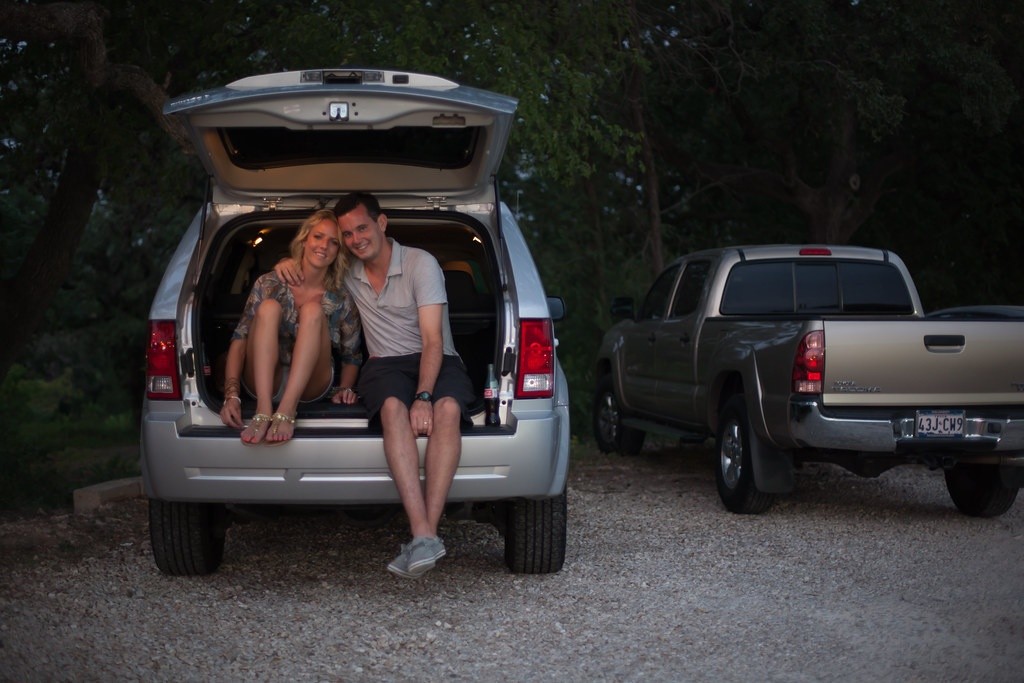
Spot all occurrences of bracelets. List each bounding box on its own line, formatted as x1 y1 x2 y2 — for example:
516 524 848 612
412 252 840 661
222 377 242 405
337 387 353 392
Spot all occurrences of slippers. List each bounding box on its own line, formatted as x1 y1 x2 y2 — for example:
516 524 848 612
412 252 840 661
264 412 297 447
241 413 270 447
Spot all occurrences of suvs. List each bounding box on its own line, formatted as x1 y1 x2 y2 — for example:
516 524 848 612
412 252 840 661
140 68 569 574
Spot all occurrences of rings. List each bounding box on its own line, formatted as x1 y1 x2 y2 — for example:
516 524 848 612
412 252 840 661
423 422 429 424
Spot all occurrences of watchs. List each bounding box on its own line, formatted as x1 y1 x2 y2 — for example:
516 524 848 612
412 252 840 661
413 391 434 401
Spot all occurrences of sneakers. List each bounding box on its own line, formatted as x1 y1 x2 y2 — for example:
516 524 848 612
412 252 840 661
408 535 446 573
386 542 435 579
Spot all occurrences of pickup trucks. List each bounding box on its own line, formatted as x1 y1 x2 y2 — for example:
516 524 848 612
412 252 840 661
596 244 1024 514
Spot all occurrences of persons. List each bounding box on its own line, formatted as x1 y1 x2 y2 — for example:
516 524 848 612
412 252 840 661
273 191 474 579
220 209 363 448
